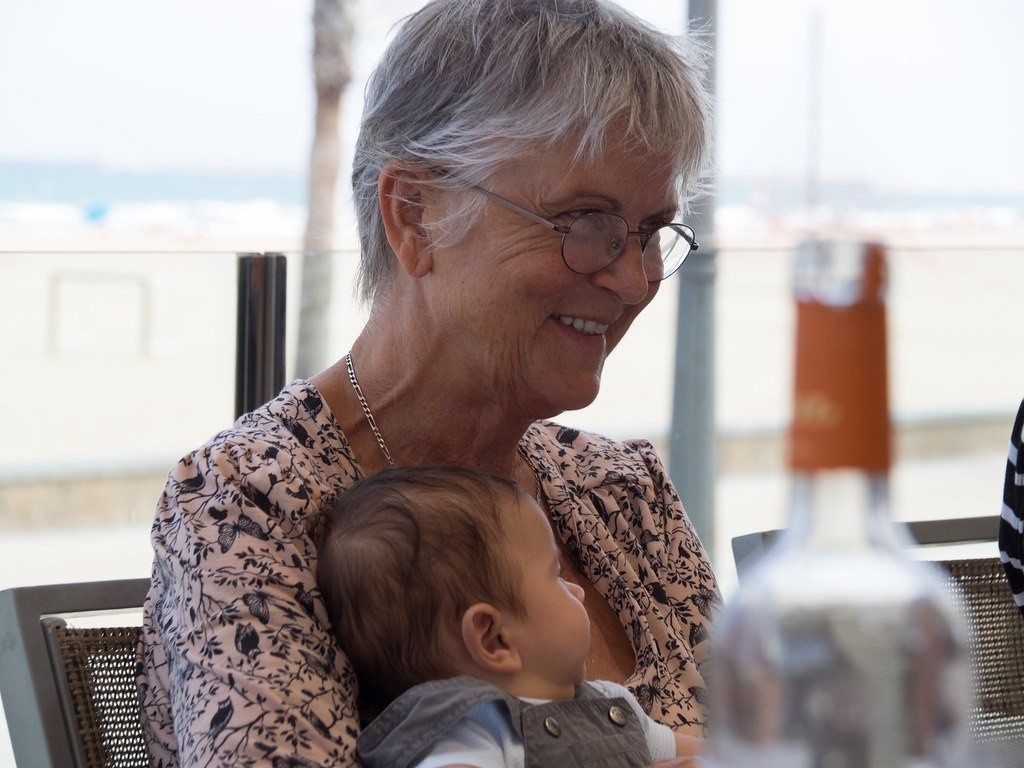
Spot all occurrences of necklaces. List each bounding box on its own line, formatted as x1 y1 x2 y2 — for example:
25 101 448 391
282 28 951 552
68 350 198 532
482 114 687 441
345 349 541 508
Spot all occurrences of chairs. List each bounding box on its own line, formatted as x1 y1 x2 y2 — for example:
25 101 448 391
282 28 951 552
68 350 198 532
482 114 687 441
0 579 153 768
730 514 1024 768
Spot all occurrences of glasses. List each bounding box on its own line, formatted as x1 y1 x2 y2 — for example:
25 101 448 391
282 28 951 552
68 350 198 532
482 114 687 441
418 161 700 284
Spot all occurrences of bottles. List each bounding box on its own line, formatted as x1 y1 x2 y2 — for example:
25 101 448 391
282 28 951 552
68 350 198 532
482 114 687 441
711 238 974 768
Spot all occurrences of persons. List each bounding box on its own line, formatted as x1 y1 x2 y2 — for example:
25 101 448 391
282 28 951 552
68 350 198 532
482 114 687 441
305 464 710 768
134 0 728 768
